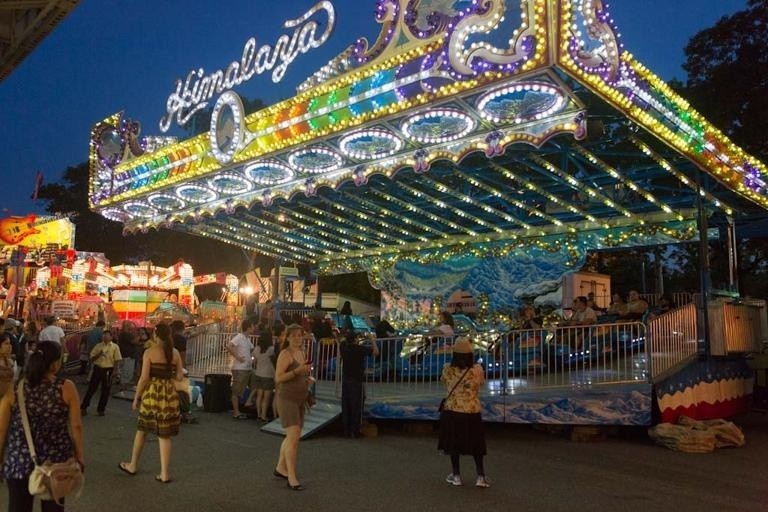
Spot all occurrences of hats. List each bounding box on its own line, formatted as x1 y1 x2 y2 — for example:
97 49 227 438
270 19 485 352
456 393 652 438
454 335 473 353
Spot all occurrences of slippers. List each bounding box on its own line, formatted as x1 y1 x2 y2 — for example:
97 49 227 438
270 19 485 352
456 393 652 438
118 461 137 474
234 412 270 423
156 474 170 483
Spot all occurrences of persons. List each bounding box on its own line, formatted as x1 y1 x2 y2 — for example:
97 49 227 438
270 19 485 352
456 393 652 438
437 336 492 488
509 287 678 343
424 311 455 345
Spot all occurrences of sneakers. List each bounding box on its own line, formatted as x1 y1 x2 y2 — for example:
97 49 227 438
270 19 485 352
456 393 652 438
476 474 494 488
445 471 463 486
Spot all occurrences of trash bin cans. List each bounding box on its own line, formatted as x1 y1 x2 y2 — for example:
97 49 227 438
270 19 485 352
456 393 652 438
205 374 232 412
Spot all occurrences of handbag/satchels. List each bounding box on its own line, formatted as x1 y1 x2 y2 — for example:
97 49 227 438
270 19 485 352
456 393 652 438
28 458 84 501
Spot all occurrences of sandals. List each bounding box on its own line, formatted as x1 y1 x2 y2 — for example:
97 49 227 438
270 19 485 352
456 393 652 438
286 478 303 490
273 467 287 479
